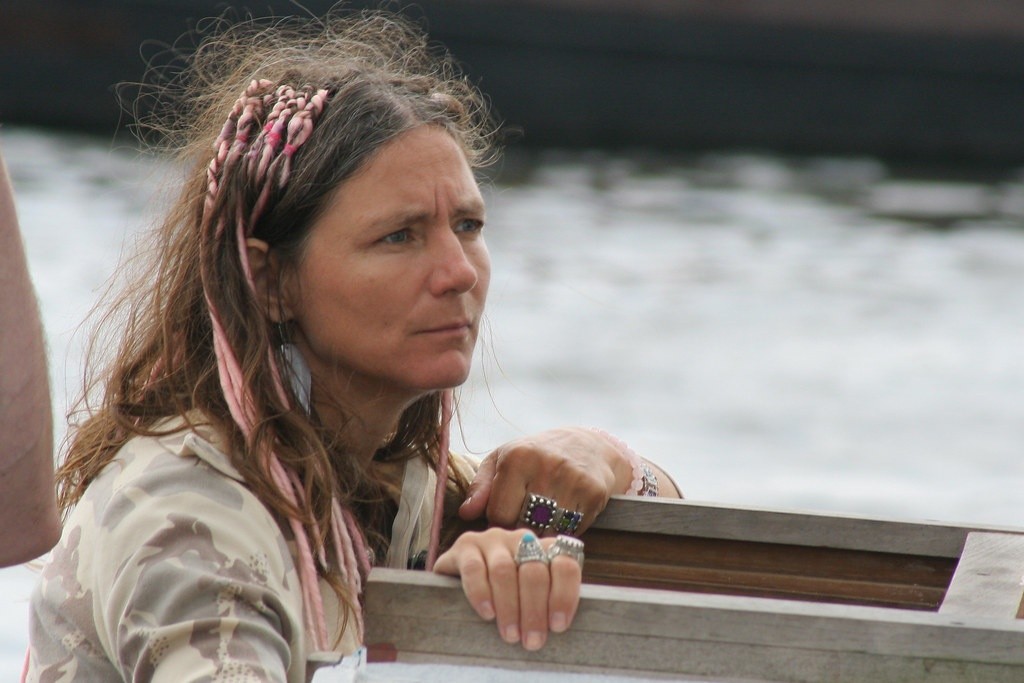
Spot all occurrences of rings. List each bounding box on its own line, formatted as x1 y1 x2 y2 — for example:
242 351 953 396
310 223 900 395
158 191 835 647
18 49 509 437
546 535 585 566
523 492 556 530
553 507 584 535
515 533 549 566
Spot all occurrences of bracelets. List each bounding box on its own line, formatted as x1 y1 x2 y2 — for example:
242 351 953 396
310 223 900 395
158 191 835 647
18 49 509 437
574 424 659 499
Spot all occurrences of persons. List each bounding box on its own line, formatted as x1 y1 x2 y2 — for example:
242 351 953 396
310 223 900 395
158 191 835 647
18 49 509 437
0 168 63 567
25 18 686 682
